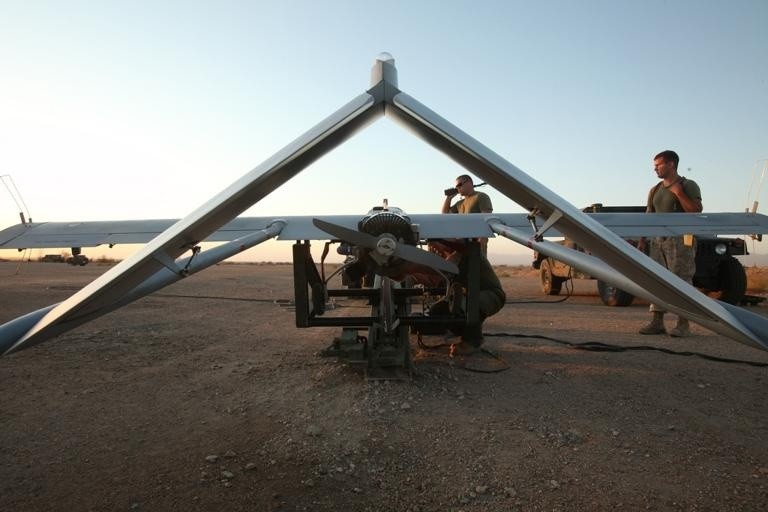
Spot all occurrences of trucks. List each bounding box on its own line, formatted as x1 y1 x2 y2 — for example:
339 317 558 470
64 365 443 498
42 254 61 263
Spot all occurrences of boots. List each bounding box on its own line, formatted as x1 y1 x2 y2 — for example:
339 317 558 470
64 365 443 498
669 315 690 337
639 311 664 335
450 341 481 356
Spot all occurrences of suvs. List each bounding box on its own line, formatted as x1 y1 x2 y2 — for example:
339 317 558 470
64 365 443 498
532 204 751 307
66 255 91 266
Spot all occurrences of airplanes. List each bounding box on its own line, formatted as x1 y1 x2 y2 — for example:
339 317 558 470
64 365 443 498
0 51 768 380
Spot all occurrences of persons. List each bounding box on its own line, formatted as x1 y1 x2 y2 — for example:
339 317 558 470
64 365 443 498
441 174 494 257
636 149 704 338
415 238 506 355
